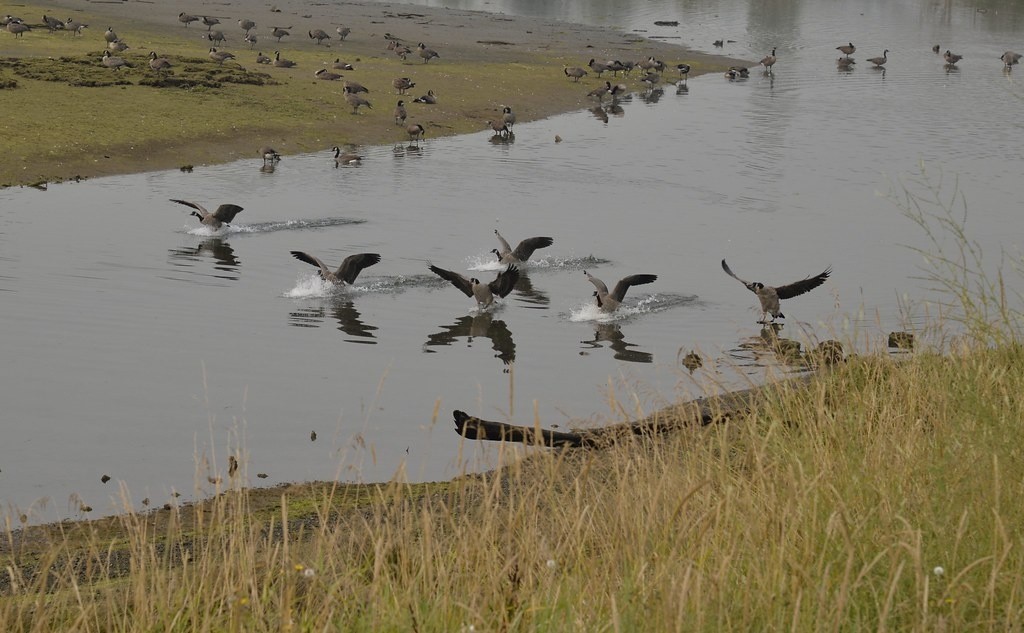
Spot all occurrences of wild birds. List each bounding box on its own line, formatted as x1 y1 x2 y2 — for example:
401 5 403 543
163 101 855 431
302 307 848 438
168 199 244 231
564 41 1024 104
163 238 243 281
287 295 379 345
489 229 553 265
509 268 551 311
578 320 654 364
582 270 658 314
290 251 382 287
720 258 835 325
425 256 520 313
0 12 515 165
419 313 518 374
